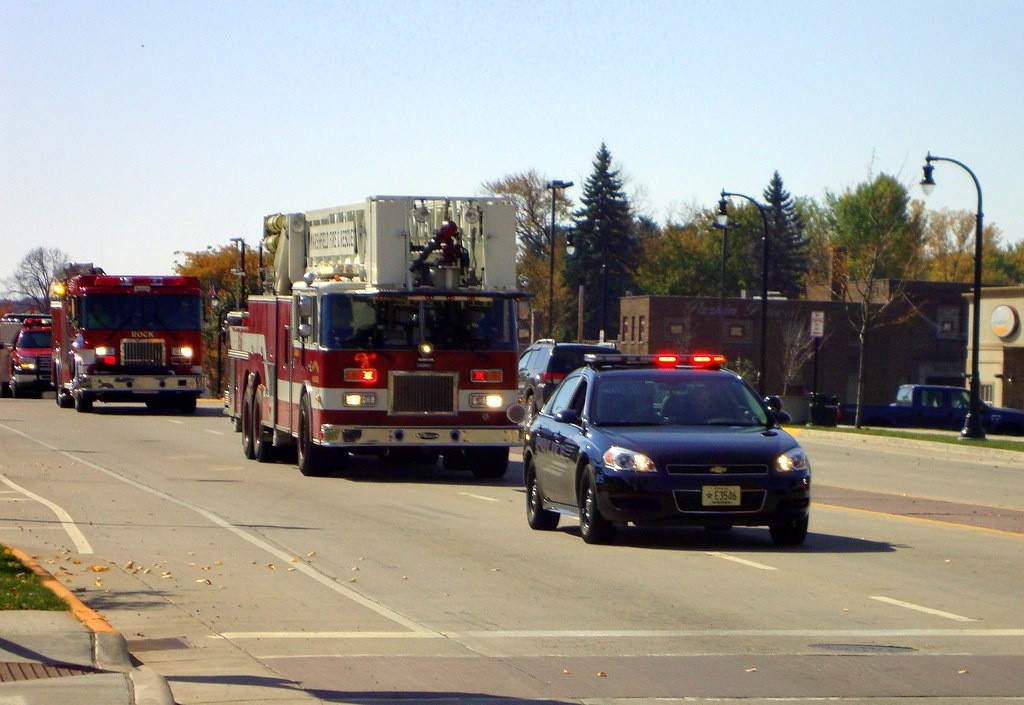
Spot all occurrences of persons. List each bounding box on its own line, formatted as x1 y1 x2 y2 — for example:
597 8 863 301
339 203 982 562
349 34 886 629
674 385 735 425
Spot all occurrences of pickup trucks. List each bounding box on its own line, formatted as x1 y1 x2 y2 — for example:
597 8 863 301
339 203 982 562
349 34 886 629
833 383 1024 437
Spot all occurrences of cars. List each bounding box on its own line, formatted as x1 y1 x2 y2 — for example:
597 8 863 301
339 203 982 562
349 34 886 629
523 351 813 549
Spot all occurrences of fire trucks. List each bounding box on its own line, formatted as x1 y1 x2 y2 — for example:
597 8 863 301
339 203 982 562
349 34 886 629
46 261 207 414
0 311 56 398
223 193 537 478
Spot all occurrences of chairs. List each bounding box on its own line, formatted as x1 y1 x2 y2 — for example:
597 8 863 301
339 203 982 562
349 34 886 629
659 393 684 422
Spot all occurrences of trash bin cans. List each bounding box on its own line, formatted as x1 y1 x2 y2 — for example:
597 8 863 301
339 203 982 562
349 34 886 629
808 391 839 428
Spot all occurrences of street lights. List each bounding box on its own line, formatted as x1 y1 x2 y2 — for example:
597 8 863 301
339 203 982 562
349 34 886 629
212 288 234 396
544 181 574 334
564 224 608 338
227 236 247 309
717 187 770 394
919 152 987 439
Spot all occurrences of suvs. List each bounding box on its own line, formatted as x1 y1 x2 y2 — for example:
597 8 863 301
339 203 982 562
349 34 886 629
519 336 634 441
